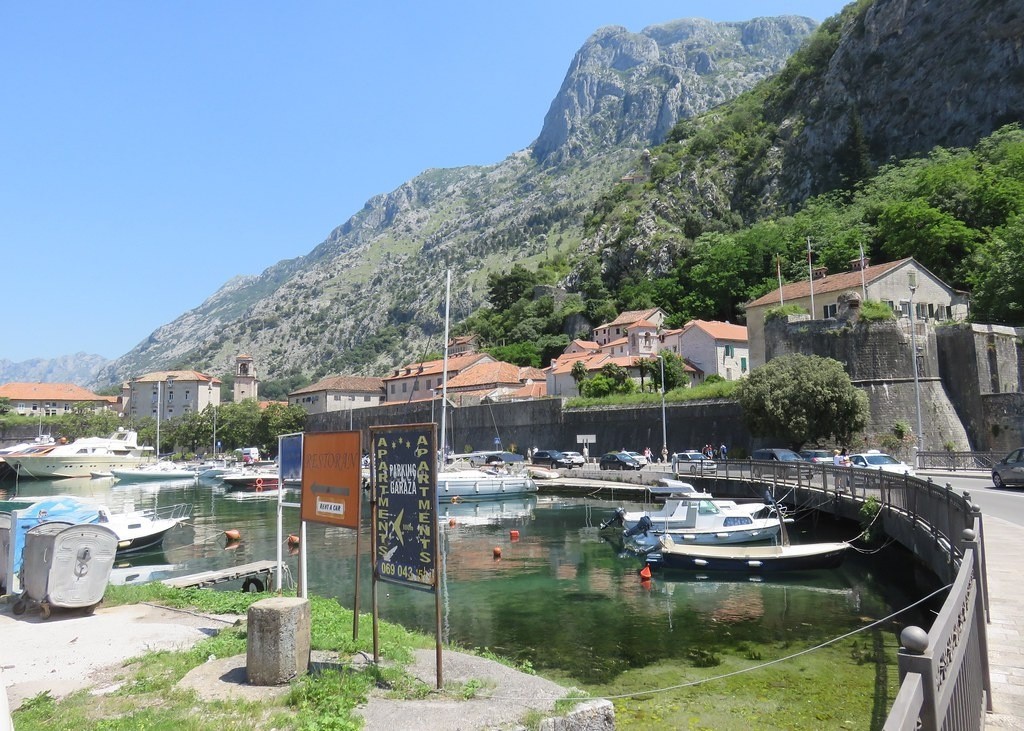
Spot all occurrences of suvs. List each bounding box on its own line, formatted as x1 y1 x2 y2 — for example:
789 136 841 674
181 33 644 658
671 449 718 474
530 449 573 469
751 448 814 481
468 450 501 468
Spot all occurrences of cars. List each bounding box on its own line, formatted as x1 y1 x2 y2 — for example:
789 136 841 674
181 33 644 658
619 450 648 468
845 451 917 487
485 453 524 466
797 450 836 471
991 447 1024 488
560 452 585 467
600 453 641 471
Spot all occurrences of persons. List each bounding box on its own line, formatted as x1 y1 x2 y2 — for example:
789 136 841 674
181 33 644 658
582 446 589 463
833 448 852 493
702 442 728 460
661 446 669 462
98 511 108 522
527 446 539 464
644 447 653 464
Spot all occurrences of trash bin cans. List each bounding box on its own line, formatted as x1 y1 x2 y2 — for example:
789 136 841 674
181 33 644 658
12 521 121 620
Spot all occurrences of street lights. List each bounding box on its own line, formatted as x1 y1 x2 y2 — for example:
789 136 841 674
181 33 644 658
639 352 667 464
906 266 926 469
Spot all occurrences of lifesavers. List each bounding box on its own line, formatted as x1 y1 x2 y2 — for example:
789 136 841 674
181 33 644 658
255 478 264 486
255 486 263 492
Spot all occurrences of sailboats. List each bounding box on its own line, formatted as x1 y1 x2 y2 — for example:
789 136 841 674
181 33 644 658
397 270 540 502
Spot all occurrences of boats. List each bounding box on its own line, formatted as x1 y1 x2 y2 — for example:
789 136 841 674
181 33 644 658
621 479 795 548
0 489 193 588
646 534 850 573
0 411 282 491
